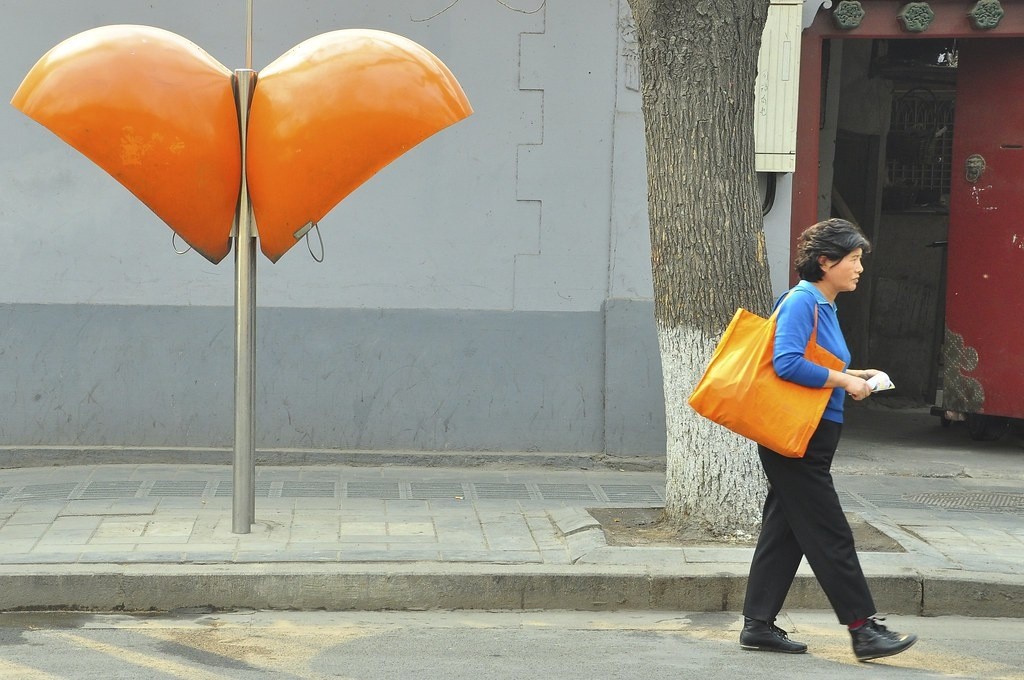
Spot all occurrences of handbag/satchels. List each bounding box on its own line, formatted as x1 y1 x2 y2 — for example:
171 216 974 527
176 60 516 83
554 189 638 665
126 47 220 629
688 288 846 459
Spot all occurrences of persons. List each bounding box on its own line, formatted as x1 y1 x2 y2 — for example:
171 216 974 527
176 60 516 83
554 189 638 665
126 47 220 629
738 218 921 661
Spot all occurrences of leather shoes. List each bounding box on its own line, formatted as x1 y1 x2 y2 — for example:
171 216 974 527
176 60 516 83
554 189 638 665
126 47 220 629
848 617 918 661
739 616 808 654
